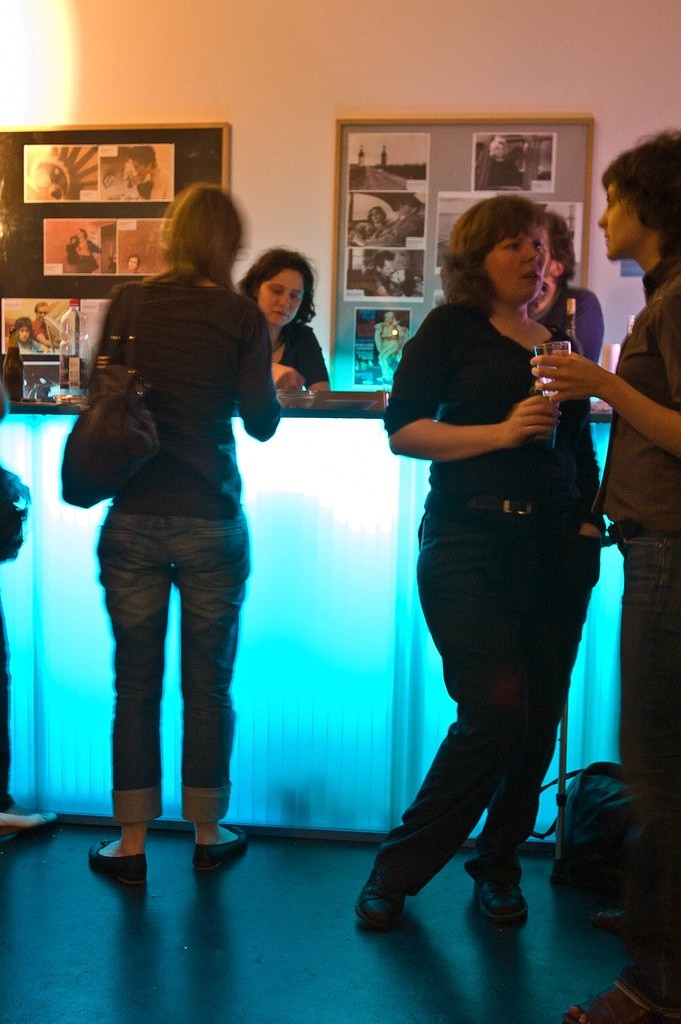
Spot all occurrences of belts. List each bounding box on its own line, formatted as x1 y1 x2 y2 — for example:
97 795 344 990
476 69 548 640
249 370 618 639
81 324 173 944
438 494 546 517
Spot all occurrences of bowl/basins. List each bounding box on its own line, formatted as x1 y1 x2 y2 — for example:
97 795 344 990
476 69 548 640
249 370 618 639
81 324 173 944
276 389 318 409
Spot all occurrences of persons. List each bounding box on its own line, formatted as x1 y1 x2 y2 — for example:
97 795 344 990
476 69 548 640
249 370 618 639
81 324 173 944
529 211 604 364
66 228 102 272
360 249 415 297
130 145 172 199
489 136 527 185
375 312 400 382
355 193 607 931
348 194 426 247
531 127 681 1024
88 182 283 882
14 301 60 352
127 254 141 274
239 248 330 392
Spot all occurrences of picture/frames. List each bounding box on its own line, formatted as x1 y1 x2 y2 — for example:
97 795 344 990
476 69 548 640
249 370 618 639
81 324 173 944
328 115 597 390
1 121 232 404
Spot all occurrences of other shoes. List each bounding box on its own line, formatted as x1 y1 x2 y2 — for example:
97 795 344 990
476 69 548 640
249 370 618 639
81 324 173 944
192 824 248 871
88 839 147 884
0 803 55 838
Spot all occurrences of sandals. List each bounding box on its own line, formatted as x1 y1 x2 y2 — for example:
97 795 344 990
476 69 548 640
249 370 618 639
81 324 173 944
561 985 658 1024
590 909 628 935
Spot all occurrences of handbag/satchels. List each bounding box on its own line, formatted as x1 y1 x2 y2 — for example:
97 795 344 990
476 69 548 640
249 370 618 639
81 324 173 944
63 283 158 506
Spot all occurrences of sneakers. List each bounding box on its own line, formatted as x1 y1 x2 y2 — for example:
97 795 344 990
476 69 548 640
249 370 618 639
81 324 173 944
355 871 403 931
475 875 529 921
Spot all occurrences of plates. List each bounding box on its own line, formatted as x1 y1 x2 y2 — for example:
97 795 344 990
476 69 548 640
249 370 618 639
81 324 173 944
326 400 378 411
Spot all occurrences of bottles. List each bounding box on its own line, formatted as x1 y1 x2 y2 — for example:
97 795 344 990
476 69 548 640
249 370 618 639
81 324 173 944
59 299 90 405
525 385 559 447
556 298 582 358
622 315 636 344
3 328 23 401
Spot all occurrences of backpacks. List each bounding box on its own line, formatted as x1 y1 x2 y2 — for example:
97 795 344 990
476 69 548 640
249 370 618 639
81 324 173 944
533 762 644 904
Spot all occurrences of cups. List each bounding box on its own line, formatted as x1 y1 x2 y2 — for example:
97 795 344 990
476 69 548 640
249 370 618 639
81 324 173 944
602 343 622 375
534 341 571 396
